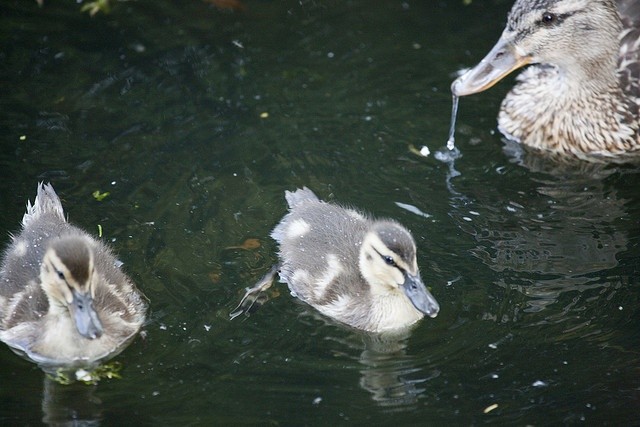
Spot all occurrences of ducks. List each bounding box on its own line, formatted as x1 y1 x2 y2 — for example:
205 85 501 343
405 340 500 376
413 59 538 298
229 185 441 333
1 181 148 374
450 2 639 165
